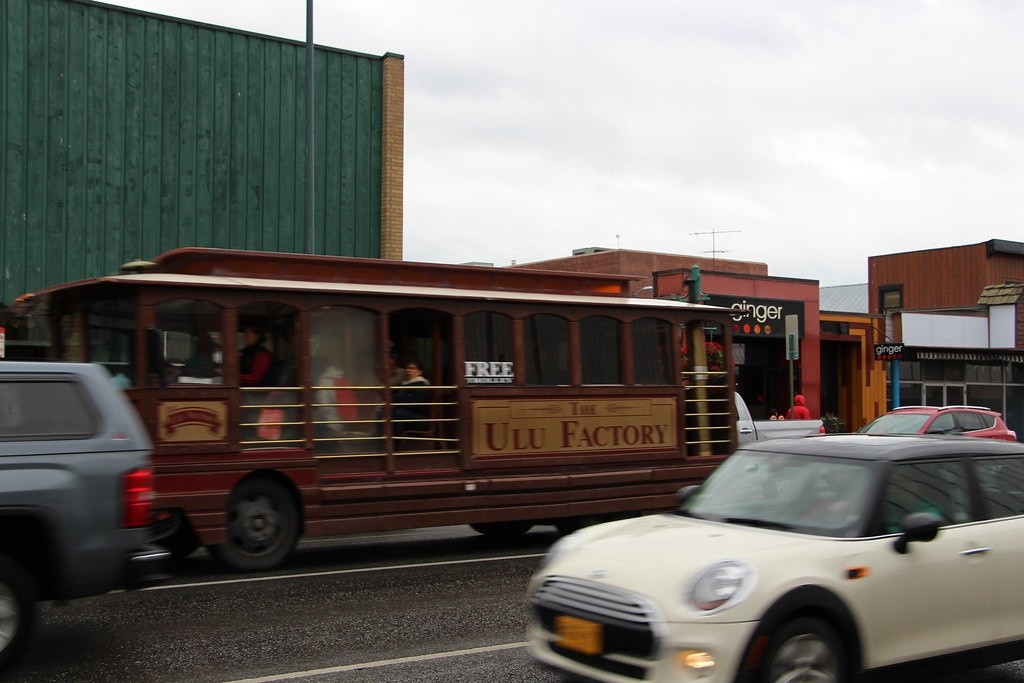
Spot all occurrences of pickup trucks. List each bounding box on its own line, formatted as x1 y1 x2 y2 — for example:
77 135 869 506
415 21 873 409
732 387 827 441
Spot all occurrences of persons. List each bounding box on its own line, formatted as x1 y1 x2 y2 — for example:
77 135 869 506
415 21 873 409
236 323 277 388
392 360 431 421
786 393 809 423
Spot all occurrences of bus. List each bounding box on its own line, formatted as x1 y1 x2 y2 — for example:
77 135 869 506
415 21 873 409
0 245 751 572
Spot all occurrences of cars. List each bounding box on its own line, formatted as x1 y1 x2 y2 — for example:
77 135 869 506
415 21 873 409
521 435 1024 682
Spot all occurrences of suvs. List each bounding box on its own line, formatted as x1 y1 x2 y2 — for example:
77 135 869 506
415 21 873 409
0 357 170 665
857 404 1017 443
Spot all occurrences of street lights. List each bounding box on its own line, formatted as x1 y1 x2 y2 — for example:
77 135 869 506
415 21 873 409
632 285 654 297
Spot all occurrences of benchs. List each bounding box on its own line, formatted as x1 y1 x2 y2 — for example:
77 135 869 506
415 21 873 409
398 402 436 451
